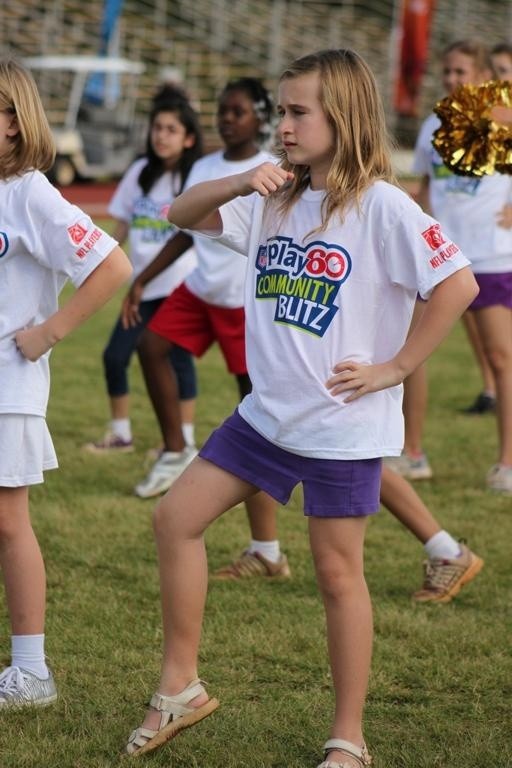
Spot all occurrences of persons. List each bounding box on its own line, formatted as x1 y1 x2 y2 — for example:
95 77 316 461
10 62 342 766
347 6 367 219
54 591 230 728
208 463 485 605
127 50 482 767
387 43 512 493
135 78 291 499
84 98 201 454
0 54 133 710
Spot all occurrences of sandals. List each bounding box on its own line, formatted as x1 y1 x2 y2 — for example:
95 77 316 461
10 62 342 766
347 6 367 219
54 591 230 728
317 739 372 768
120 678 219 759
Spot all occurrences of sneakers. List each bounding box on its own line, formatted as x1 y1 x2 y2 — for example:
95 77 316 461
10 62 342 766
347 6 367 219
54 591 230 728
382 452 432 478
209 551 292 580
411 537 485 604
81 432 134 454
0 666 57 711
134 446 199 498
